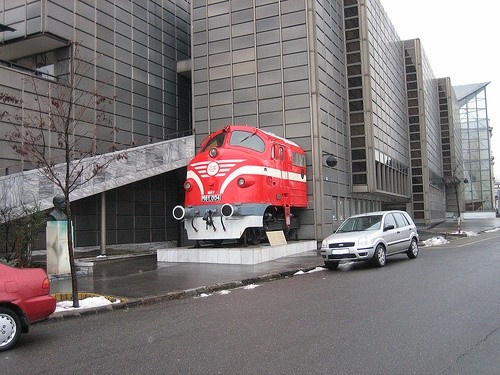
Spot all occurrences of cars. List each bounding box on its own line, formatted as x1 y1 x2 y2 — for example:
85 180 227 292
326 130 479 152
1 260 58 354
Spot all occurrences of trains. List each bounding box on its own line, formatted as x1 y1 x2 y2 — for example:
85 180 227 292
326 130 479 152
172 123 308 248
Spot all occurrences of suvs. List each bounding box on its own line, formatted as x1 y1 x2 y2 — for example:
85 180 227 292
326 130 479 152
320 209 420 271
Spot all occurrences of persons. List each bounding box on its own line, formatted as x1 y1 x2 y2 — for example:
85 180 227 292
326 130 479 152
49 194 67 221
